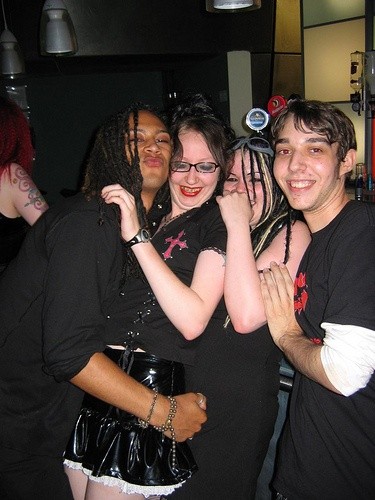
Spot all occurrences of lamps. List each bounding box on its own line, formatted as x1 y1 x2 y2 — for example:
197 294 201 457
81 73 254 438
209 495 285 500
0 30 25 79
40 0 78 58
206 0 262 13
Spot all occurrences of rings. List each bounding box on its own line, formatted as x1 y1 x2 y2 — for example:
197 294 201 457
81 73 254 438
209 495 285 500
196 393 204 405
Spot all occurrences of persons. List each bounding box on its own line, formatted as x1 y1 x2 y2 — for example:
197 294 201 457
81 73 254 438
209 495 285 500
0 92 50 280
163 135 313 500
0 99 208 500
61 100 234 500
256 100 375 500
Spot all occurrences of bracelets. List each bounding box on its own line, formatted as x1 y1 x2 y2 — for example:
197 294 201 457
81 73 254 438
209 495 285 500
152 392 179 472
137 389 158 429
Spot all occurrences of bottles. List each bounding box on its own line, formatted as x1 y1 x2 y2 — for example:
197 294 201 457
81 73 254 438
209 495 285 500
364 50 375 115
351 52 365 94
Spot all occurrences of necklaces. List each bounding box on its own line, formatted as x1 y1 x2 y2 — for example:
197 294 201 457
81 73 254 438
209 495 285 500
159 210 189 232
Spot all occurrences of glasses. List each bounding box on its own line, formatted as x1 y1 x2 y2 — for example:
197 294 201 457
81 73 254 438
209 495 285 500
169 161 219 173
225 136 274 157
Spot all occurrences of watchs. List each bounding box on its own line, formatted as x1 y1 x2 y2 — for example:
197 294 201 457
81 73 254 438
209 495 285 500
124 229 150 248
